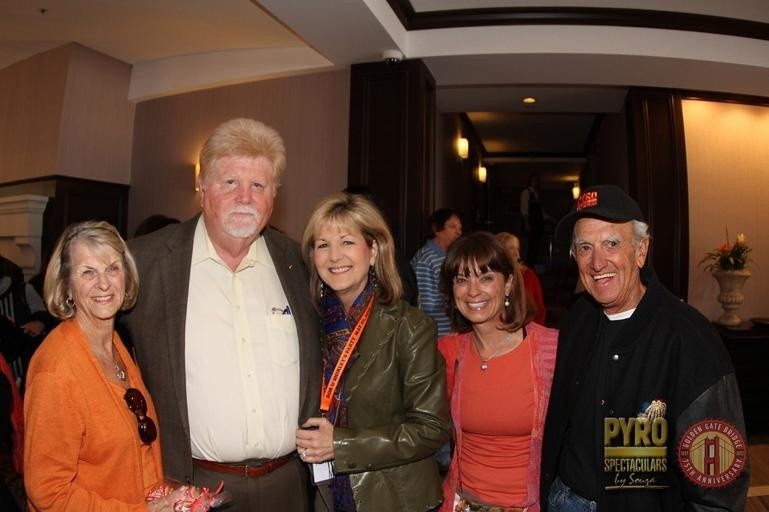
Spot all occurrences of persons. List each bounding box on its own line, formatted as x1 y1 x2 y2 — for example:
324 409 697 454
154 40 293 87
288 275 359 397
436 231 559 512
294 191 451 512
409 207 471 335
546 184 749 512
2 118 323 512
494 232 547 327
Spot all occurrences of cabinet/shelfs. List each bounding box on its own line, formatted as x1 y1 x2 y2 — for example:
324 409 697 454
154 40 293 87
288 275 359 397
712 321 769 443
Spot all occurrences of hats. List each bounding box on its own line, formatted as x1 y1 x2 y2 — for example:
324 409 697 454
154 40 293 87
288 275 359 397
555 184 643 247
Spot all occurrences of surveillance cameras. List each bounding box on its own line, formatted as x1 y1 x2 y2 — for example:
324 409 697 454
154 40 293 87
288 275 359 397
383 50 403 68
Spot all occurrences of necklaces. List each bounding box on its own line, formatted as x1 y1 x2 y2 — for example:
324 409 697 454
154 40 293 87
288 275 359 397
471 334 514 375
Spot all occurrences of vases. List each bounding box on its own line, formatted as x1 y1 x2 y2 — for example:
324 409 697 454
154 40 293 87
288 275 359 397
711 270 751 325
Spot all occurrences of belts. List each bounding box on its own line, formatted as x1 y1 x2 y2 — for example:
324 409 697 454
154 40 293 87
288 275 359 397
192 455 293 479
456 489 527 512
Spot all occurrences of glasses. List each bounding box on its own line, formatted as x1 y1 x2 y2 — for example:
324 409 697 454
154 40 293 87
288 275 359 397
124 388 157 443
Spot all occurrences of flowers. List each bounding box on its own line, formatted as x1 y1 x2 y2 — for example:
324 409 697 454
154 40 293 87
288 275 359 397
696 223 760 274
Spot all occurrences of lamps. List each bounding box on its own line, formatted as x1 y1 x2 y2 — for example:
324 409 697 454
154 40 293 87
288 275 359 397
479 166 487 182
457 137 469 159
